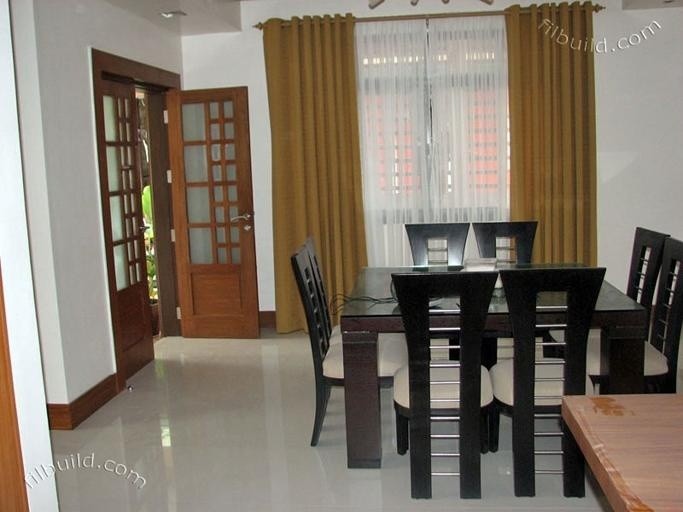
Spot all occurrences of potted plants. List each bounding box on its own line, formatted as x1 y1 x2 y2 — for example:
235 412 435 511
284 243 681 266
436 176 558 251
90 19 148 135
135 184 162 336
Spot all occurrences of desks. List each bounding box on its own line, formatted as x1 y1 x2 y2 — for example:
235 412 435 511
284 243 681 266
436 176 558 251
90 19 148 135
559 394 683 512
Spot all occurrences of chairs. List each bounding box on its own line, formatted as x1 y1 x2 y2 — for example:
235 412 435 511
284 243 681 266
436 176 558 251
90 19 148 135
471 220 549 372
290 246 409 456
391 271 498 500
306 241 407 343
542 227 671 359
402 223 470 360
491 268 607 498
585 236 682 393
